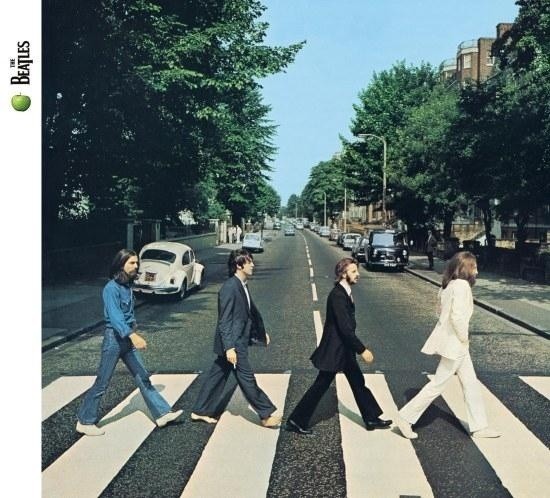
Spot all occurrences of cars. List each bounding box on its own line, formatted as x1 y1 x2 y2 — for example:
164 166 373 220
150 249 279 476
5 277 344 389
242 233 264 252
284 225 295 235
272 221 281 230
131 241 205 301
294 218 368 263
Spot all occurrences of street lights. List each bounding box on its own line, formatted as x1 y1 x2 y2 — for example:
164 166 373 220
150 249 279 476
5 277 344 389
358 132 387 227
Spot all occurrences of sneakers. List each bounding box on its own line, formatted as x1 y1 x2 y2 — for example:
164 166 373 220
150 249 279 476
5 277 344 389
260 416 282 427
155 409 184 427
392 413 418 439
190 411 218 424
473 429 501 438
76 420 105 436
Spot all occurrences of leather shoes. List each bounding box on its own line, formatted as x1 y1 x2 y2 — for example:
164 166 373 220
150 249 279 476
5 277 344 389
366 418 392 430
286 418 313 435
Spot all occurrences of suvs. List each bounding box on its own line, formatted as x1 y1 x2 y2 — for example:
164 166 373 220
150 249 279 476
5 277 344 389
364 228 414 272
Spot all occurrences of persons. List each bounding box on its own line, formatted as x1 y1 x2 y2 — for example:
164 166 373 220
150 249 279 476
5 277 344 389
192 249 285 428
76 248 184 437
236 224 242 244
288 258 395 433
395 251 501 439
427 230 437 271
